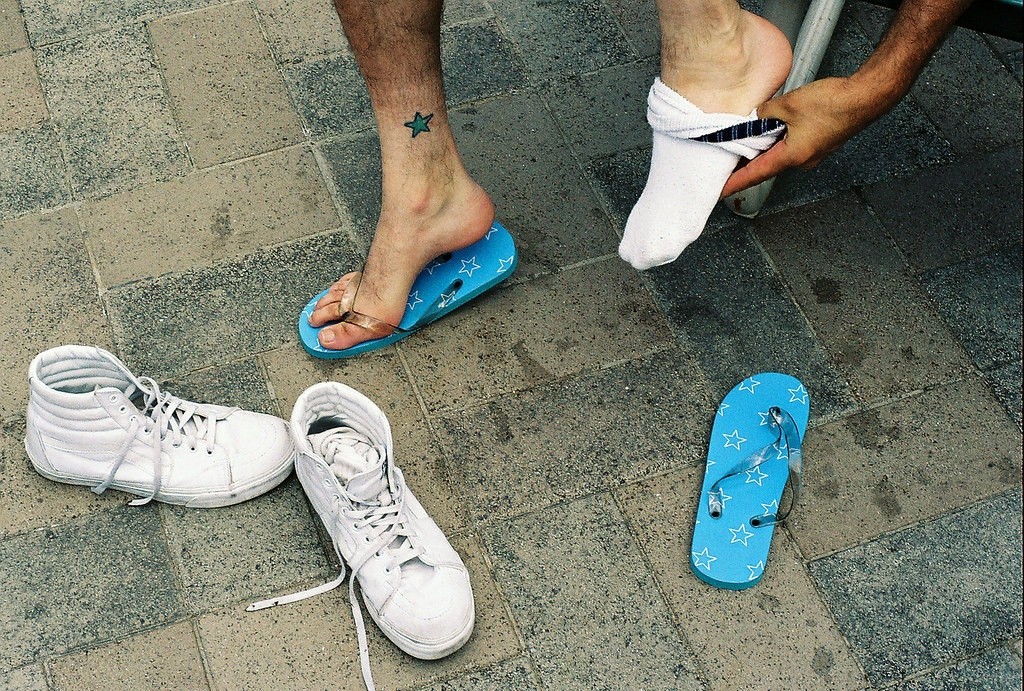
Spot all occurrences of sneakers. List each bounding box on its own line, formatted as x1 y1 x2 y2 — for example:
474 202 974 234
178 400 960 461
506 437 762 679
245 382 474 691
24 346 294 508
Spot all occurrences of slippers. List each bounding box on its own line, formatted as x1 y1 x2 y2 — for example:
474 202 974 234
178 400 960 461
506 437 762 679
690 373 810 592
297 221 518 360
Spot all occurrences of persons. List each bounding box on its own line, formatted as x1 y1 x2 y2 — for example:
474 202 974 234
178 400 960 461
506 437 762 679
306 0 960 349
